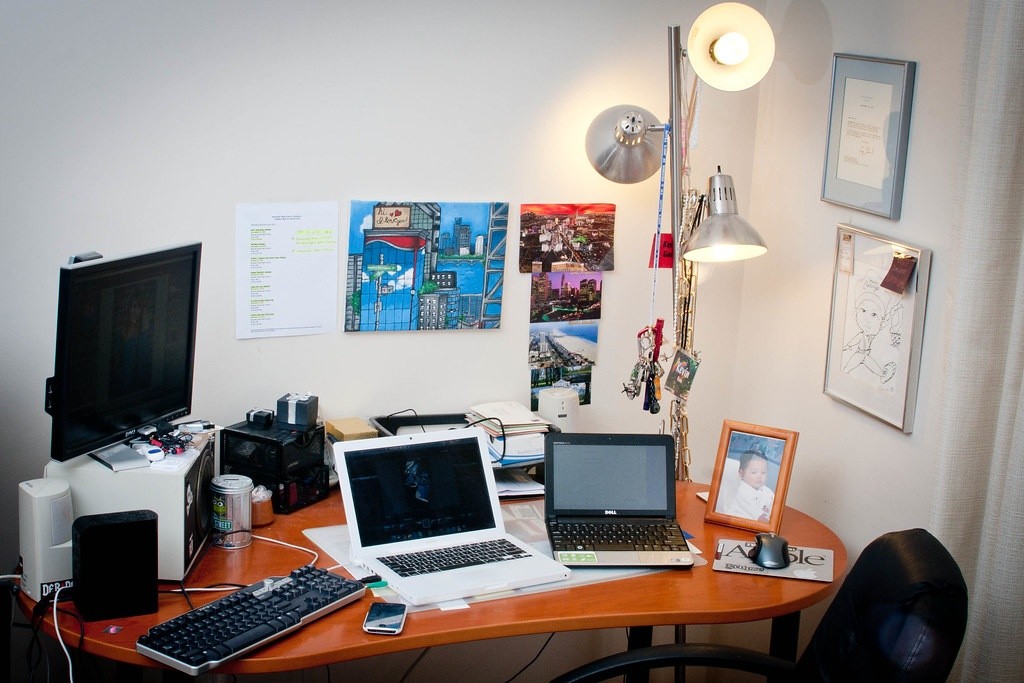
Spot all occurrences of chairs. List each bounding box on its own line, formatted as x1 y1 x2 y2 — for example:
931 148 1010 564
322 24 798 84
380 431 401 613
548 527 969 683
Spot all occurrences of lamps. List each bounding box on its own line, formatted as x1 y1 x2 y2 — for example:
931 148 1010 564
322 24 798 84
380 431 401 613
586 0 777 482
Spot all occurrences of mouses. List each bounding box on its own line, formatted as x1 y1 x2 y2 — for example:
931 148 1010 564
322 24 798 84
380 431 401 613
749 532 790 569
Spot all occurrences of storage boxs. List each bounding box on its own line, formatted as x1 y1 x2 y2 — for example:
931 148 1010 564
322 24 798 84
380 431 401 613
277 393 318 432
324 418 379 441
247 407 275 430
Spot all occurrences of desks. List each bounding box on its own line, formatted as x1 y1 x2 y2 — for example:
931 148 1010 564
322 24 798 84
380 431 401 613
10 480 848 683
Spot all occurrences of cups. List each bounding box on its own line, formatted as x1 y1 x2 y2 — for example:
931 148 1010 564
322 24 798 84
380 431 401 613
210 474 254 549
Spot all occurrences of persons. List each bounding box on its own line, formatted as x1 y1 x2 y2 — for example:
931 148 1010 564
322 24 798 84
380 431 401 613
667 357 691 387
731 451 774 521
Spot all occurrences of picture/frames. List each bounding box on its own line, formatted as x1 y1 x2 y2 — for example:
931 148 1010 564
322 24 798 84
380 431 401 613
822 222 933 435
820 51 917 221
705 419 799 535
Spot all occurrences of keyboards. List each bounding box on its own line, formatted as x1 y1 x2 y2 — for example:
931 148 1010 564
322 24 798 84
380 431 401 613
137 564 366 675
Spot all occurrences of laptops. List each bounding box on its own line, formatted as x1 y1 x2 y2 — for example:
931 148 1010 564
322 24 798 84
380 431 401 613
543 432 694 569
332 428 572 605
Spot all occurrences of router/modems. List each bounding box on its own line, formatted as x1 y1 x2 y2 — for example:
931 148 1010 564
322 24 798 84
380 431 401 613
72 508 160 622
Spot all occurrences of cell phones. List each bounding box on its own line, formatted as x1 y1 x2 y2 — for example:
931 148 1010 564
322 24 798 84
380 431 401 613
362 602 407 635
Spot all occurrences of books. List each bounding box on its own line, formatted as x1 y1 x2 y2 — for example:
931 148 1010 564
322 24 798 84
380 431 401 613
469 401 548 468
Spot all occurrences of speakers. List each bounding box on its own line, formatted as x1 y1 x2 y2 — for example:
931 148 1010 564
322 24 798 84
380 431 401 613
18 478 78 603
44 431 216 582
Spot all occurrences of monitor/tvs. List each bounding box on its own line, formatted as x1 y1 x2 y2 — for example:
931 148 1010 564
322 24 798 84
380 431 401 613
44 242 203 471
